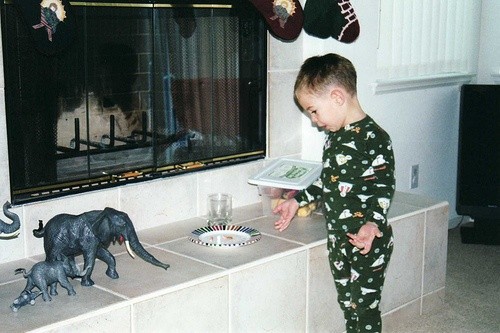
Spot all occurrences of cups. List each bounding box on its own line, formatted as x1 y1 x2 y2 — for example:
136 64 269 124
206 193 233 226
257 185 283 217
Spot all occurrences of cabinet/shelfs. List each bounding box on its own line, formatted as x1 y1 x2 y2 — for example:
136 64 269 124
0 191 451 333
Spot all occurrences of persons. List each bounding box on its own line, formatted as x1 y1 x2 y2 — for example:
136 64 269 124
273 52 394 333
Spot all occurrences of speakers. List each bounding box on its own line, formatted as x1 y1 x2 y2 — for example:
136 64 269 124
456 84 500 244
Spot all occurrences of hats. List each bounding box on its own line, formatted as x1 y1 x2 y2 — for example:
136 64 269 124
304 1 360 44
250 0 305 42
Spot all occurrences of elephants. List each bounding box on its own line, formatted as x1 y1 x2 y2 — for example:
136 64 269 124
0 200 170 312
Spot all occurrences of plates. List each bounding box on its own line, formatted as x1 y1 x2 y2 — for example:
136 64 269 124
188 225 262 249
